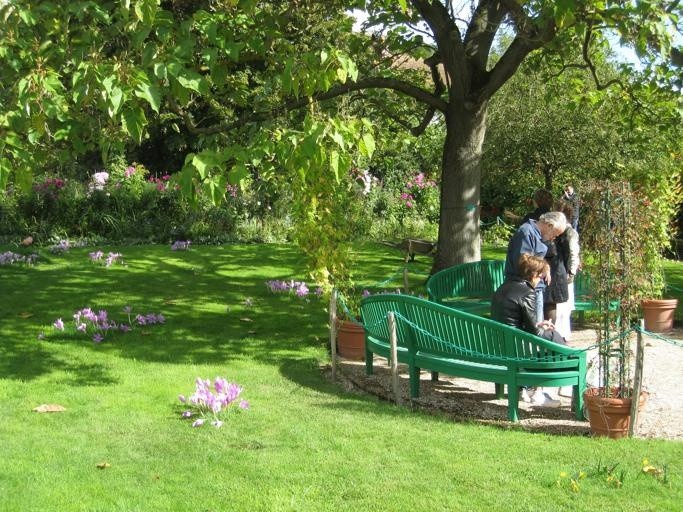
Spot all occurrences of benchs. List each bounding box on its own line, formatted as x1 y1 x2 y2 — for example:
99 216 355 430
357 293 589 424
423 256 623 328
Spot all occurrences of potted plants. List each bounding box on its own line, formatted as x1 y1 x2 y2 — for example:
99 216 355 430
584 184 649 438
632 186 678 333
309 187 367 361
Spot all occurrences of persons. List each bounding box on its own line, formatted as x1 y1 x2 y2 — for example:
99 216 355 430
489 252 570 412
560 185 582 228
553 201 583 342
504 208 568 326
518 187 571 329
